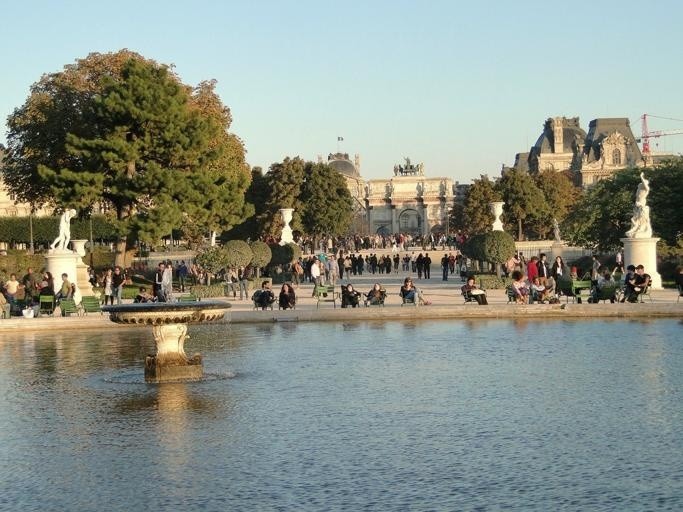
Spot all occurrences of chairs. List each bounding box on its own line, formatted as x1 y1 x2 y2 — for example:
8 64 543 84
461 287 487 305
315 285 341 309
570 279 595 304
595 277 619 305
398 286 423 306
504 286 523 305
0 280 104 320
365 288 387 307
673 278 683 303
179 289 199 302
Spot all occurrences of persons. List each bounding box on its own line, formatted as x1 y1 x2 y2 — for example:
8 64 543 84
132 252 249 302
0 265 125 318
252 279 273 310
675 264 683 298
50 209 76 249
625 172 652 238
341 283 360 307
287 230 464 298
362 283 384 307
398 278 430 306
278 282 295 310
461 276 488 305
403 156 409 167
504 248 649 305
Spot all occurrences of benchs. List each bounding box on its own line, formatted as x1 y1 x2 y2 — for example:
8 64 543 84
619 272 654 305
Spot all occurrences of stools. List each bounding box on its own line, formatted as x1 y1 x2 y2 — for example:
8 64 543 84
252 300 273 312
341 296 361 309
277 300 296 310
